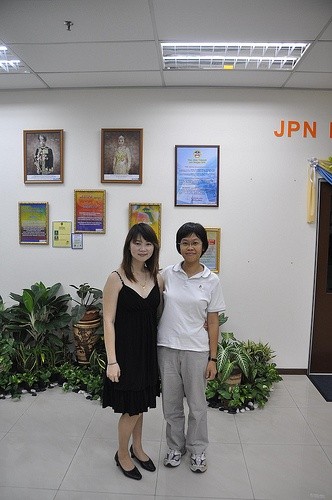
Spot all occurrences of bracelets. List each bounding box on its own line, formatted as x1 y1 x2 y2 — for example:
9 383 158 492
210 358 217 363
107 363 117 365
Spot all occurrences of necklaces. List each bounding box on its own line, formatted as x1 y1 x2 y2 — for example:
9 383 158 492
135 280 151 289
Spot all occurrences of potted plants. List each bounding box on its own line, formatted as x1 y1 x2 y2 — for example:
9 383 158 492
216 331 253 412
67 283 106 365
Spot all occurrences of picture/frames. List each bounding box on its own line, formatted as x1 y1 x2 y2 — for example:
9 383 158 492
174 145 220 208
23 129 63 184
18 201 49 245
73 190 106 234
128 202 161 249
197 228 221 273
100 127 143 184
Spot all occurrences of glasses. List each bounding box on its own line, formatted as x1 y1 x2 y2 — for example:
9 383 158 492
179 241 203 246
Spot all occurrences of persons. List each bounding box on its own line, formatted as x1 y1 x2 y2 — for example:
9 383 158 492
160 222 226 473
112 136 131 174
33 133 53 174
100 224 165 480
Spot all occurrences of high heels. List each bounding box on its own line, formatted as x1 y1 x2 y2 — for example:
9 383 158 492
115 450 143 480
129 445 156 471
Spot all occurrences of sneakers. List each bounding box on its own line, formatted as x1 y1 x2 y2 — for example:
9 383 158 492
191 451 208 472
163 445 187 466
